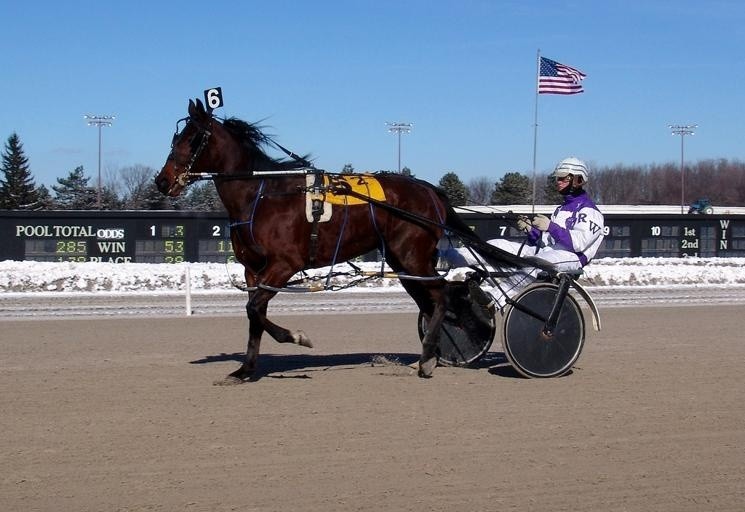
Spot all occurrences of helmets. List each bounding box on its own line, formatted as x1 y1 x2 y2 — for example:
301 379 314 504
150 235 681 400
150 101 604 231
552 158 589 184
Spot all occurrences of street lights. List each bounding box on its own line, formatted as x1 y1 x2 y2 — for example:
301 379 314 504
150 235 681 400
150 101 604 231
83 113 116 210
667 123 700 214
380 117 416 176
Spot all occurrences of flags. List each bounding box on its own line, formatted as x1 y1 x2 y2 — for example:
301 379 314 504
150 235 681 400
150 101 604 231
538 58 587 95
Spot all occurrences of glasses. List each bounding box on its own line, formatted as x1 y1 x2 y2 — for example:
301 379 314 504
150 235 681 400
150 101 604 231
558 176 570 181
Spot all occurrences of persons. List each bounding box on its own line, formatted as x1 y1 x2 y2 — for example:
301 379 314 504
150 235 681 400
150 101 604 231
441 157 605 312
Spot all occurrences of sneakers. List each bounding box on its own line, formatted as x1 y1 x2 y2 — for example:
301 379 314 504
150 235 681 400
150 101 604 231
469 279 496 318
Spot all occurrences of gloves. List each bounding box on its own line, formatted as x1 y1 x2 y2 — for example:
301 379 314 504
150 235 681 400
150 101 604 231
533 215 552 233
518 216 531 232
